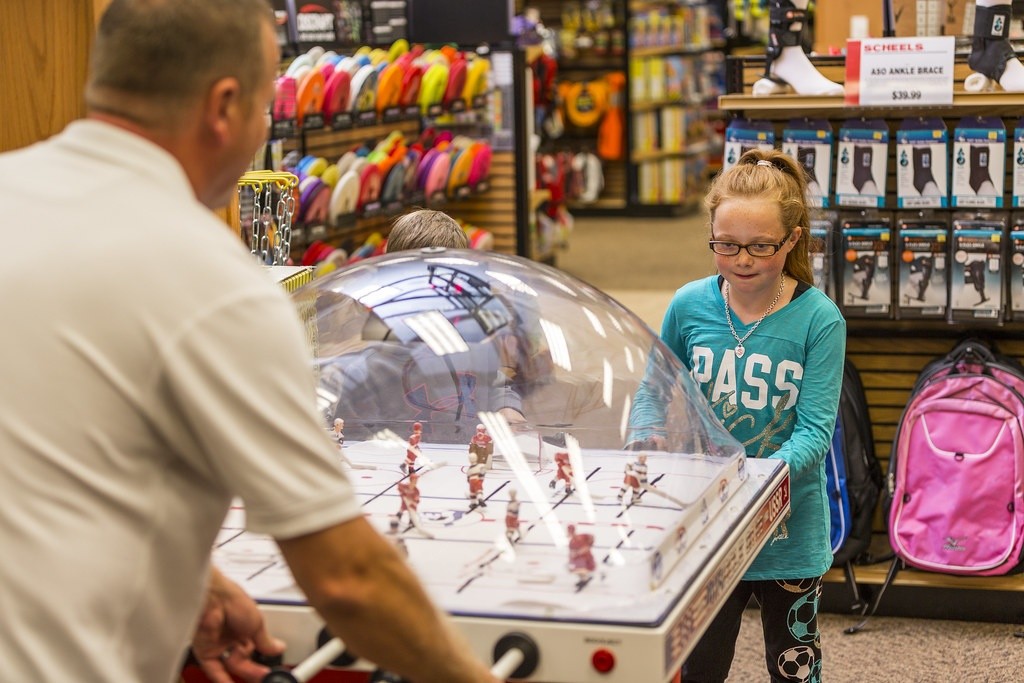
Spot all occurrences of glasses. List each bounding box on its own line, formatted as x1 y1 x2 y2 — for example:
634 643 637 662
709 211 792 257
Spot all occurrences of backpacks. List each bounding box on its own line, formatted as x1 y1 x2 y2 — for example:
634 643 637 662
825 363 883 569
893 339 1024 576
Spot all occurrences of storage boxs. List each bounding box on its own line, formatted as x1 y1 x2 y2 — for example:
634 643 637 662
723 113 1024 328
844 34 956 109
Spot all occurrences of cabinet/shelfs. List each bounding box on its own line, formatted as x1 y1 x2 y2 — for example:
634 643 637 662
257 0 734 270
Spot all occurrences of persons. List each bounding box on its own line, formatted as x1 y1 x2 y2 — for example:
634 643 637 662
621 149 847 683
549 450 574 494
762 0 1024 97
325 208 528 445
0 0 505 683
399 422 423 474
325 417 345 444
468 424 494 470
390 474 420 533
617 452 649 504
465 452 492 509
565 525 597 586
504 487 524 543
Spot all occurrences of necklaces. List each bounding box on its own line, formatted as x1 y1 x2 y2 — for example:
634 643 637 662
724 273 786 359
